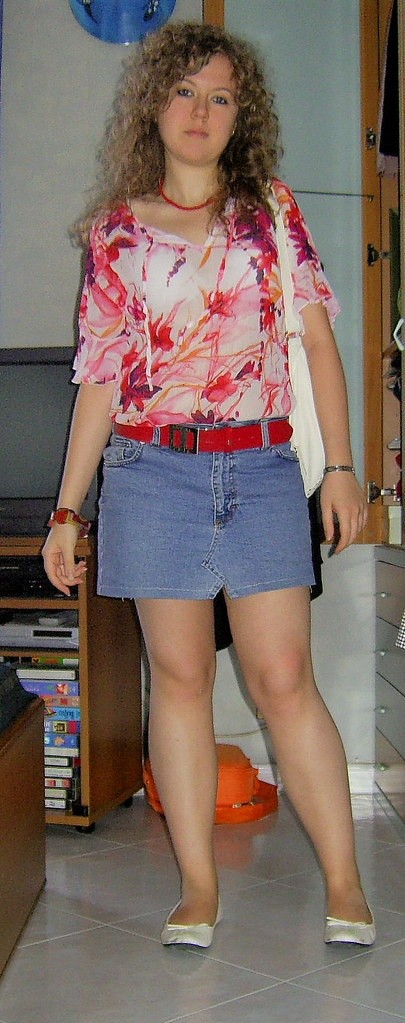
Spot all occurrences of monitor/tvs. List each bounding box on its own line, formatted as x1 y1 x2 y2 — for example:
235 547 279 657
0 347 104 538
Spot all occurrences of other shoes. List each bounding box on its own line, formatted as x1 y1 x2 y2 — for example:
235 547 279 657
324 894 376 946
161 891 222 949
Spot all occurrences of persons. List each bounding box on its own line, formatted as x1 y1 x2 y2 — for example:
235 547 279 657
42 23 376 947
54 723 64 731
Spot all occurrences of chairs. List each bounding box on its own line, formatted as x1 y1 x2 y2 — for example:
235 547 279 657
0 662 48 985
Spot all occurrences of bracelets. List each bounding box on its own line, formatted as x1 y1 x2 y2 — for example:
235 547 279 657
323 465 355 474
47 509 91 537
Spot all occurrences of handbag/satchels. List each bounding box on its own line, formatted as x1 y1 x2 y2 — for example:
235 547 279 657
285 327 326 499
143 746 278 824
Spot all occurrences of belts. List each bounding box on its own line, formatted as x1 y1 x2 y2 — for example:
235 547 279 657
112 421 293 456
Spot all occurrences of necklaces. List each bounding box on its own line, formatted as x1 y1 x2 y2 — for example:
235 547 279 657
160 177 213 211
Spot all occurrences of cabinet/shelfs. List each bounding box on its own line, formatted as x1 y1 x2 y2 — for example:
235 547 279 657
374 547 405 838
0 535 144 834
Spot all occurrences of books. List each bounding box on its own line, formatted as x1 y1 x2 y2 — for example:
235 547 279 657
6 657 80 813
